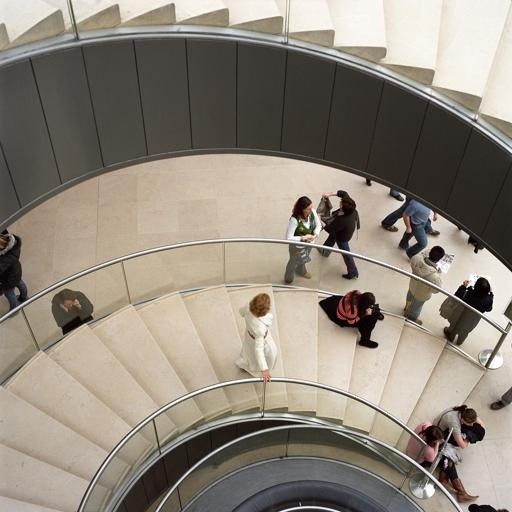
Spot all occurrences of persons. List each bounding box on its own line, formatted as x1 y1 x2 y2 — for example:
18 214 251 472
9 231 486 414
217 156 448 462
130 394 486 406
315 188 362 281
403 244 444 329
467 500 509 511
1 228 28 316
356 174 486 261
283 196 322 287
318 289 385 349
428 405 487 449
443 265 495 346
491 387 512 411
405 420 480 503
236 290 282 385
49 288 94 336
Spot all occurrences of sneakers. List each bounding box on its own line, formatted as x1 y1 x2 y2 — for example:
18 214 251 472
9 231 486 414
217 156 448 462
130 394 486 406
304 271 312 278
16 295 30 302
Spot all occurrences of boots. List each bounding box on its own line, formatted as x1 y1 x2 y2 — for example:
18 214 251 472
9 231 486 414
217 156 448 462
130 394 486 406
439 470 458 495
452 479 479 504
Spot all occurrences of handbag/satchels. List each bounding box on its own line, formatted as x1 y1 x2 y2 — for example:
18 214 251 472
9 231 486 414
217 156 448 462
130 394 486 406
440 295 465 327
292 248 311 267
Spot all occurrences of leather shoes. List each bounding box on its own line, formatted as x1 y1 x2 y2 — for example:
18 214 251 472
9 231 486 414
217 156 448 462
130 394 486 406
359 339 378 348
394 195 404 201
444 327 454 342
491 401 505 410
429 229 441 235
366 178 371 186
381 224 398 232
415 319 423 325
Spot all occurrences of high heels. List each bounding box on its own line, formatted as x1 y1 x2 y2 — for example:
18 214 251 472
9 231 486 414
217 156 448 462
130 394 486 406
342 273 359 280
